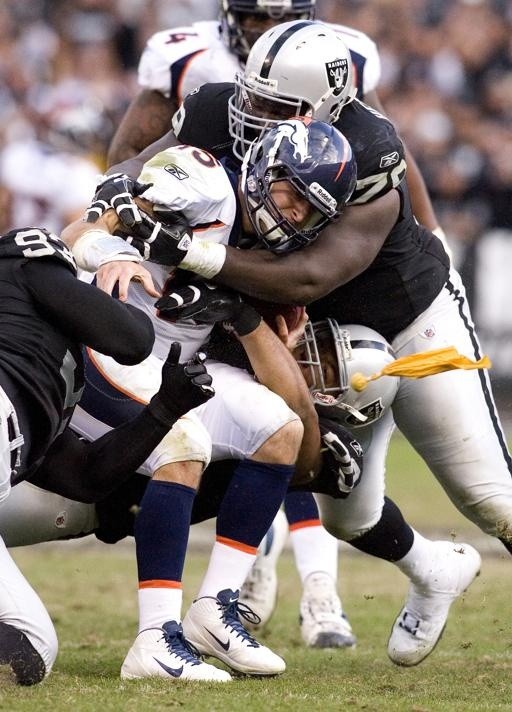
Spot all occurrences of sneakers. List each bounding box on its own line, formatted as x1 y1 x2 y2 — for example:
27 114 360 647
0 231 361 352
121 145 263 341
182 588 286 675
237 509 290 629
386 540 482 668
299 584 357 651
119 621 230 683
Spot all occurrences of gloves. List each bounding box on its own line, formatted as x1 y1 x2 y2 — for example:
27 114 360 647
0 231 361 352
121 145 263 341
155 278 263 338
83 172 226 280
148 341 216 427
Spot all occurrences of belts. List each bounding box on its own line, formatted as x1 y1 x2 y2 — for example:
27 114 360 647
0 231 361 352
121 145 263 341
7 416 18 471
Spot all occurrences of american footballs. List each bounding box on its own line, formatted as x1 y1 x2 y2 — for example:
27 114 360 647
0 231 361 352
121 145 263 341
284 306 302 332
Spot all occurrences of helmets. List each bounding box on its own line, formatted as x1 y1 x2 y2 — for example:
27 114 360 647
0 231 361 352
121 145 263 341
217 1 358 222
313 316 400 429
